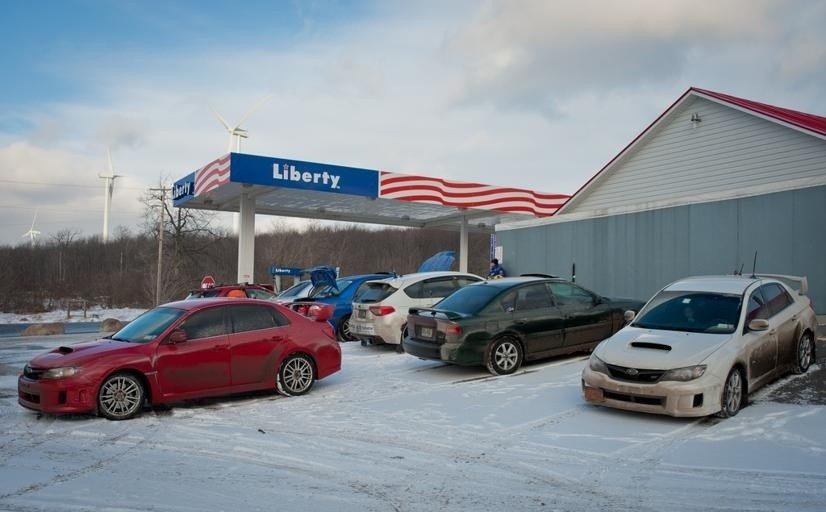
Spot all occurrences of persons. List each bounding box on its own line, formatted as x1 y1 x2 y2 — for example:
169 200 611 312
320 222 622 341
488 258 504 276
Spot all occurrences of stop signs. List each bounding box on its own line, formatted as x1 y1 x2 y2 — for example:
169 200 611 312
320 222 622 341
201 276 215 288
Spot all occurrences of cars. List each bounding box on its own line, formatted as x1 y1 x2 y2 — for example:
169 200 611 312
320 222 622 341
581 272 817 417
402 274 645 375
349 271 487 351
273 250 455 342
